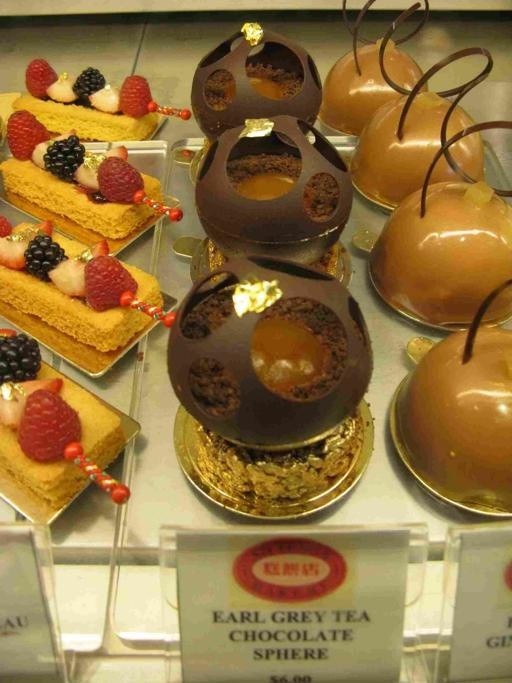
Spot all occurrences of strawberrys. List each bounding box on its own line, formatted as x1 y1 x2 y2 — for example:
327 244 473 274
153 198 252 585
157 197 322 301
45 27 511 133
0 213 12 238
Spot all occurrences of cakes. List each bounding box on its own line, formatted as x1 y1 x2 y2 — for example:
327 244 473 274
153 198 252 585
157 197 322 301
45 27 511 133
354 49 494 206
372 122 512 327
393 280 512 515
0 329 130 525
320 1 429 134
0 111 182 253
167 253 373 516
190 22 323 145
0 59 190 143
192 115 354 278
0 213 175 353
